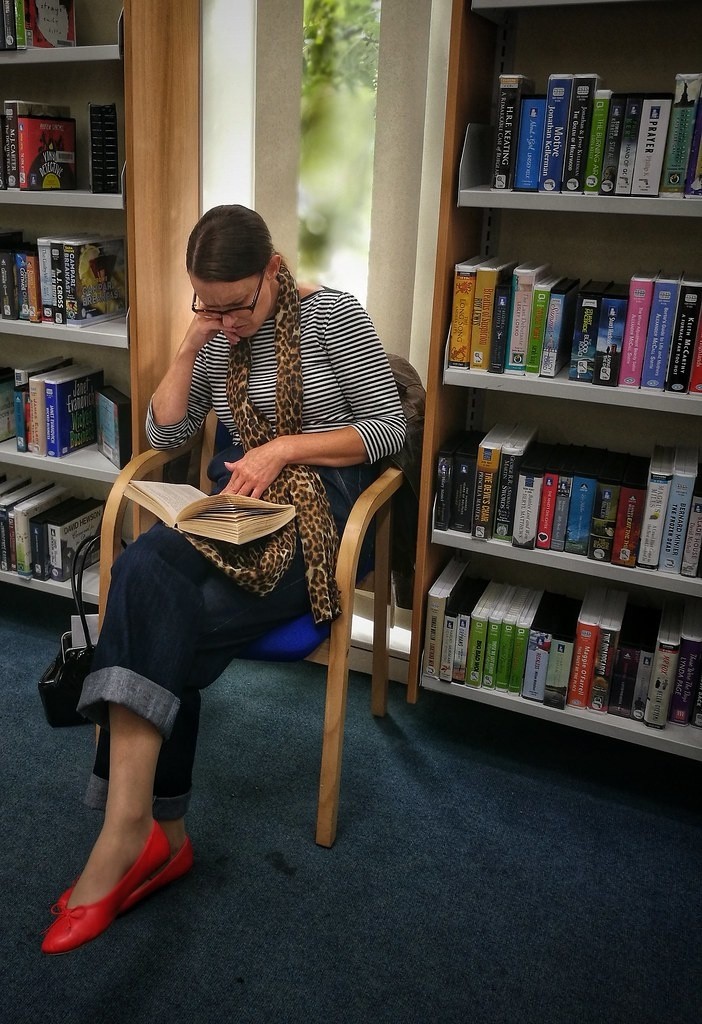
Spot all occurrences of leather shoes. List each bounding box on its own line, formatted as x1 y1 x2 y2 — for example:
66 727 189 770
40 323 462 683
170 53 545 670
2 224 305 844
39 816 172 954
55 833 193 913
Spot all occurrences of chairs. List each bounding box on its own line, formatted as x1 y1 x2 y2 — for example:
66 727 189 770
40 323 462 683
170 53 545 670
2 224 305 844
94 351 426 848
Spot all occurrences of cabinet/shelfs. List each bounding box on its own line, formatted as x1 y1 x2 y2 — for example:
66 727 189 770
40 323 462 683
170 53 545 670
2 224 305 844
407 2 702 763
0 0 204 607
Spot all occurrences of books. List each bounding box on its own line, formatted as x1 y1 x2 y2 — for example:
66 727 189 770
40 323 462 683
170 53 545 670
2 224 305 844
490 73 702 200
0 226 126 330
122 479 298 546
0 98 77 192
0 0 76 51
88 100 118 194
0 473 106 582
447 253 702 396
417 406 702 731
0 355 133 470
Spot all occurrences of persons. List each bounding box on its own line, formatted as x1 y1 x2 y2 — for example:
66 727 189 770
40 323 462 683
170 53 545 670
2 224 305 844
41 204 407 955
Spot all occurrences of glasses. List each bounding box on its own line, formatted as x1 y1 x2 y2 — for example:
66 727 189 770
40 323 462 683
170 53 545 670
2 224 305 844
192 257 266 320
